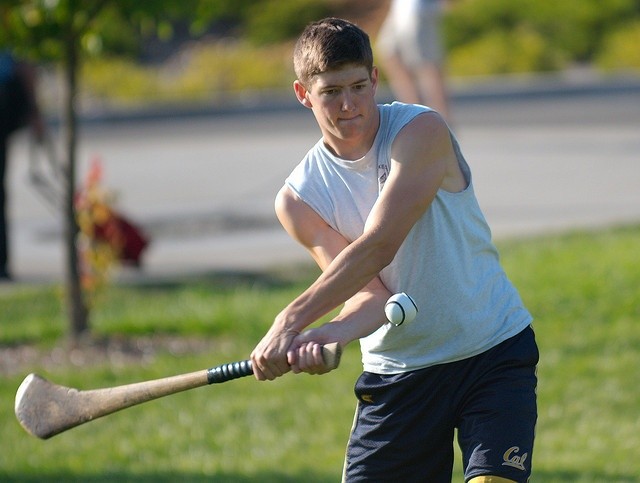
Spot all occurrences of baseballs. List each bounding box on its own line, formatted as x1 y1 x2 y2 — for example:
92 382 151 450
384 292 418 326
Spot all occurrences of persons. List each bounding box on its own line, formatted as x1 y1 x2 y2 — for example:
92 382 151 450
0 53 47 285
374 0 449 127
249 15 539 483
